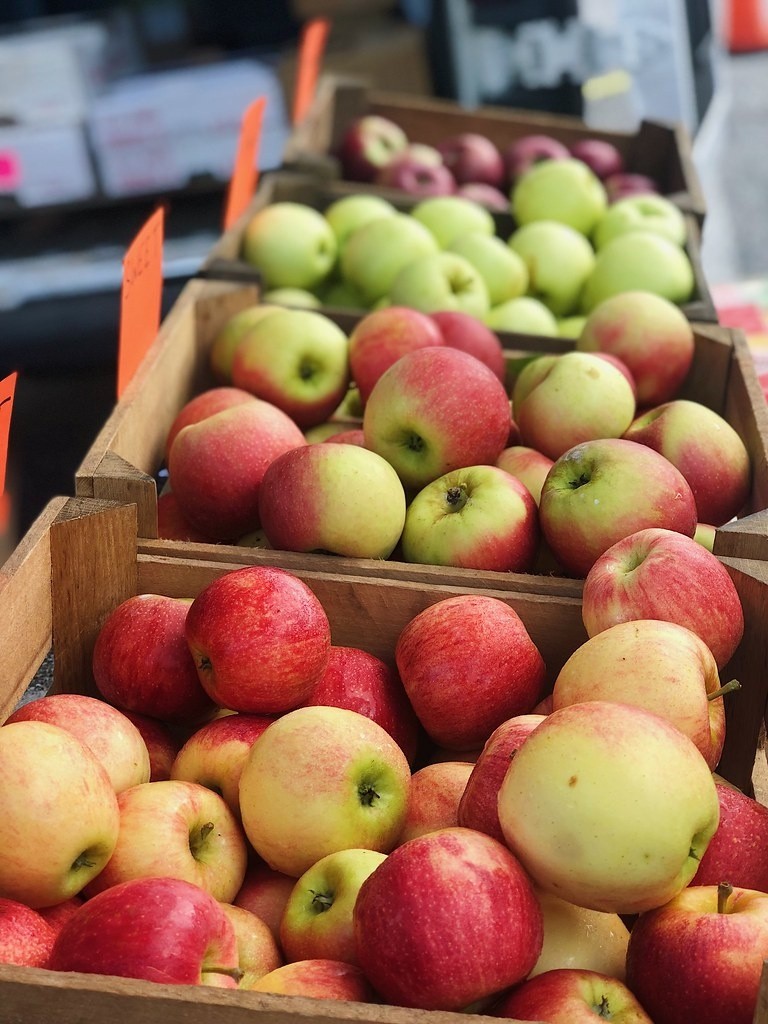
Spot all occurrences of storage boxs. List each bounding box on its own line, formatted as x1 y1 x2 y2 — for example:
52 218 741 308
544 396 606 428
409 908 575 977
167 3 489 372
1 82 768 1024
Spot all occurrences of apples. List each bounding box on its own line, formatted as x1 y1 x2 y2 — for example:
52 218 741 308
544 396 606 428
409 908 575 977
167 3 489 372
0 526 768 1024
147 298 748 577
244 158 692 340
339 113 661 208
